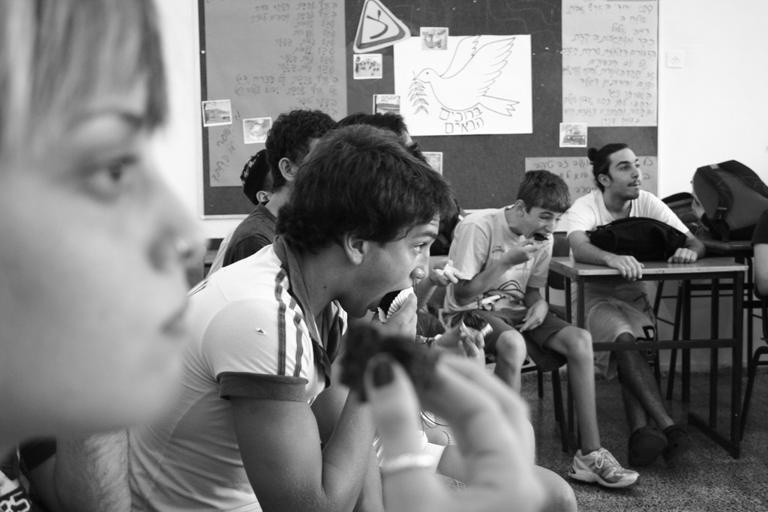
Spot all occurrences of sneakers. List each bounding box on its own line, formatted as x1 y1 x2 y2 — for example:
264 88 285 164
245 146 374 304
567 446 640 489
628 423 694 466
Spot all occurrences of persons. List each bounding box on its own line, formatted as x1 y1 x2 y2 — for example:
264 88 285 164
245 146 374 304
126 119 577 512
1 2 536 512
205 107 460 448
559 142 707 472
439 169 640 491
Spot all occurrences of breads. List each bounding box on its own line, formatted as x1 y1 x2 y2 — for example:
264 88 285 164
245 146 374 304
463 310 488 331
533 234 548 240
341 325 424 403
379 290 401 315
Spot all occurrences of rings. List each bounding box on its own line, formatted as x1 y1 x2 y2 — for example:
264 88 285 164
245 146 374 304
375 448 435 476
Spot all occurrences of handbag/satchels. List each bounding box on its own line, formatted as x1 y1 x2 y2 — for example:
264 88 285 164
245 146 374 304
589 217 687 262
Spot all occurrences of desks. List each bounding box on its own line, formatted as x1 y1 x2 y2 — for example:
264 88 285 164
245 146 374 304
548 257 749 464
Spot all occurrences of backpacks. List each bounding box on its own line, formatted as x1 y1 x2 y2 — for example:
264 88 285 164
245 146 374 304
690 159 767 244
